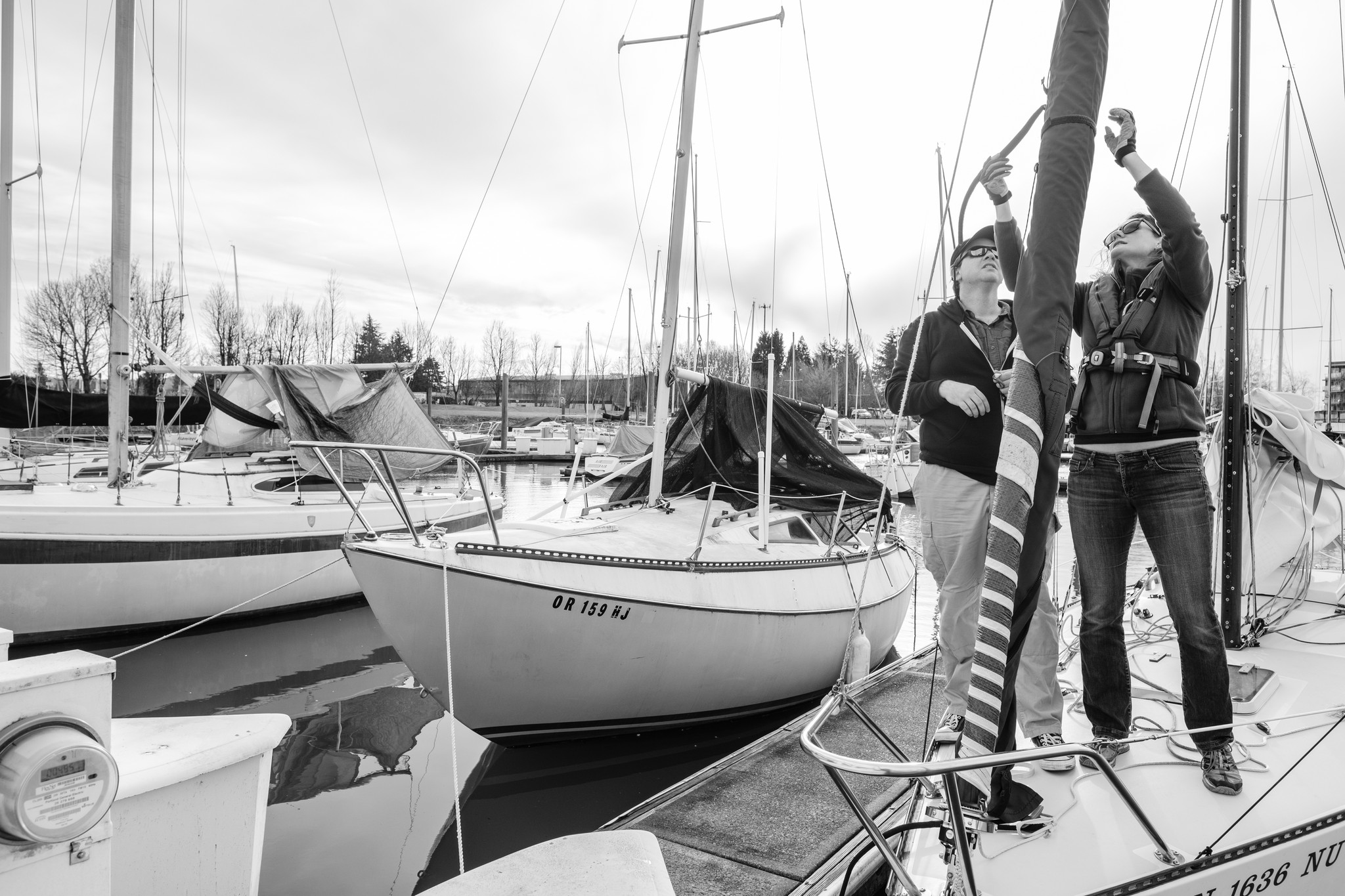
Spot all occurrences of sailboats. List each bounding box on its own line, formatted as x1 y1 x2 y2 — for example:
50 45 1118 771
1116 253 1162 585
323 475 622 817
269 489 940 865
0 0 1344 896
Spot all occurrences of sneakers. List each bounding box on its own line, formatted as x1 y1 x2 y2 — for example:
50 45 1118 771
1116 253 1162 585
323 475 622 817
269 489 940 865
935 714 966 744
1032 733 1076 771
1079 735 1130 769
1197 742 1243 795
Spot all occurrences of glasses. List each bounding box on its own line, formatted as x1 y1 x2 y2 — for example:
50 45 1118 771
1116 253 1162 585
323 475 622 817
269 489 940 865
956 245 998 267
1102 218 1162 250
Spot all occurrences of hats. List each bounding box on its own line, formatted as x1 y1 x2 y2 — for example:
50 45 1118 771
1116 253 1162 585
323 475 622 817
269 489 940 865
950 225 995 268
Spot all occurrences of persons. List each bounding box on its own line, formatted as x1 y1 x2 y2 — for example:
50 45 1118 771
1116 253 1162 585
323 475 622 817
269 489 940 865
889 223 1076 774
982 110 1243 796
